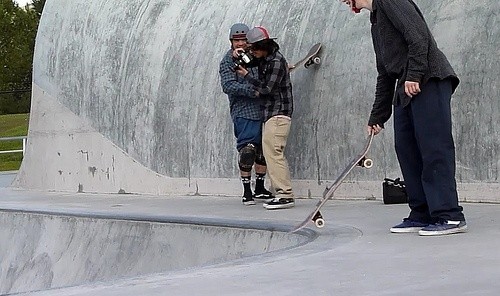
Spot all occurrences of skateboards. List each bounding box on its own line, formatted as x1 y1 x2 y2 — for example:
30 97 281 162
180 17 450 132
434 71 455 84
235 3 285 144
289 43 322 73
288 129 374 234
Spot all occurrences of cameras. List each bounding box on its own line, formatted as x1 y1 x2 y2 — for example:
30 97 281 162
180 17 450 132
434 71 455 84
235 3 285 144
229 51 253 72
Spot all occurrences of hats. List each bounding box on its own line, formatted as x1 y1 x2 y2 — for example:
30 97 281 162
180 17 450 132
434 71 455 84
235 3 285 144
246 27 277 44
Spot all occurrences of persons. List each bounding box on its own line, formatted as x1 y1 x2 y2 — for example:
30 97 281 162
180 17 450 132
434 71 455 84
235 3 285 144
342 0 469 236
220 23 275 206
235 26 294 210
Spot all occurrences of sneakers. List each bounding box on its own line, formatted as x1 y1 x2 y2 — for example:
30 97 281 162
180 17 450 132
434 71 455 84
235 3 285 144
263 197 295 209
242 195 255 204
419 219 467 235
390 218 427 233
254 188 275 198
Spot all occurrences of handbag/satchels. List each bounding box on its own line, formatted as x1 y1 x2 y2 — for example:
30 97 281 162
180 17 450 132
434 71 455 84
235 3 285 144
383 178 408 204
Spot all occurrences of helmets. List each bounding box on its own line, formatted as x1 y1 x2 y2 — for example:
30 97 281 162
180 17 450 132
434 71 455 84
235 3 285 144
229 23 249 40
